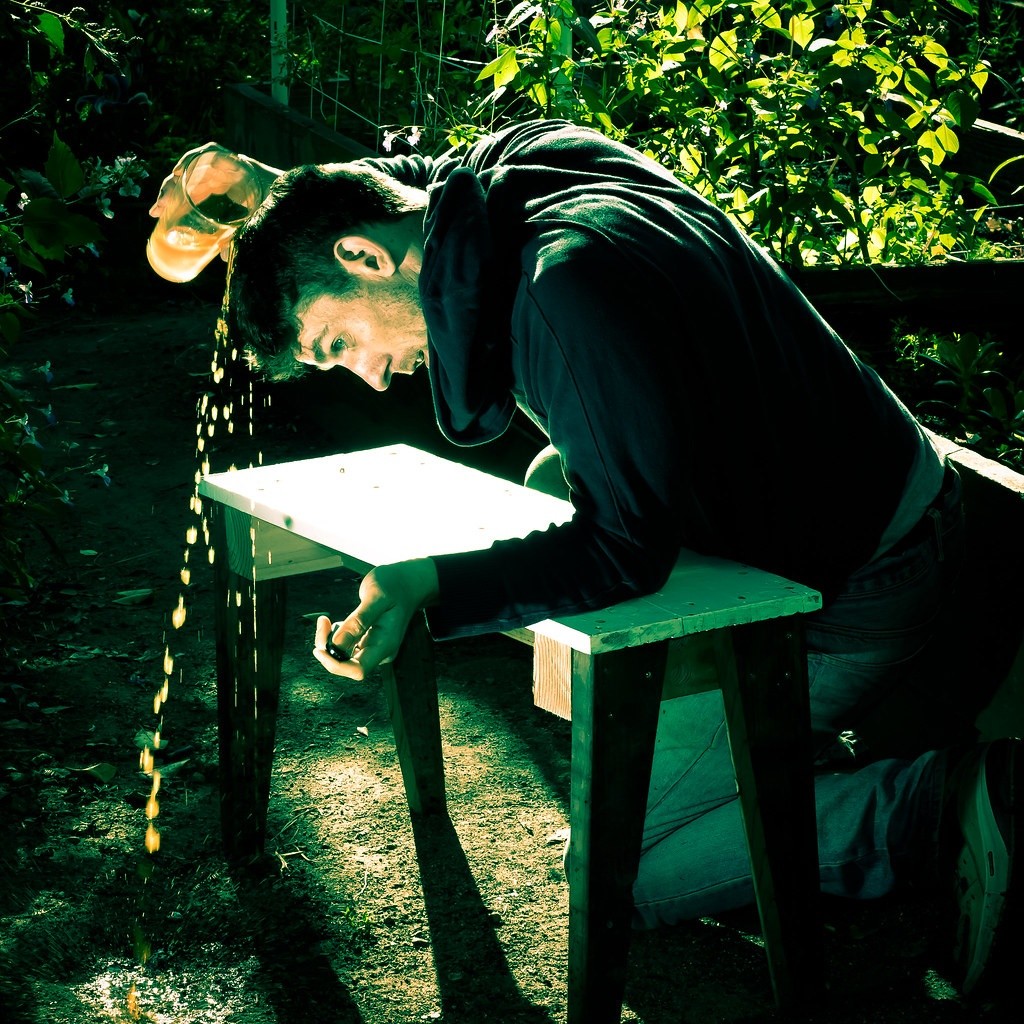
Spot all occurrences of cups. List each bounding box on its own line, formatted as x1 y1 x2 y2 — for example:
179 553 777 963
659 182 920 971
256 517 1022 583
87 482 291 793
144 149 262 284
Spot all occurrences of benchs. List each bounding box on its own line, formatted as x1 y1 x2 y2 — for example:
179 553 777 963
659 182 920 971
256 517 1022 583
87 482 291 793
199 445 824 1024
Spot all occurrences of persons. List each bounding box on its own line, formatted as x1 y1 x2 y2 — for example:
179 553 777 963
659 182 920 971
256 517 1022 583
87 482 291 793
148 117 1024 996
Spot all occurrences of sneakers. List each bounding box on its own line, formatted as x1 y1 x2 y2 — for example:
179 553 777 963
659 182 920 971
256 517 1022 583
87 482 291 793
933 737 1024 994
